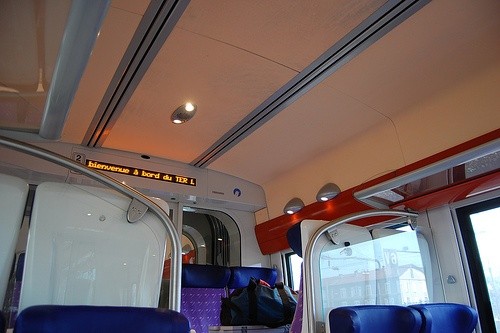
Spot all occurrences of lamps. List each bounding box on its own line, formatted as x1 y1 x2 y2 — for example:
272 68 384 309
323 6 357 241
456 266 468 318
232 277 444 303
171 103 197 124
283 197 304 214
316 184 341 202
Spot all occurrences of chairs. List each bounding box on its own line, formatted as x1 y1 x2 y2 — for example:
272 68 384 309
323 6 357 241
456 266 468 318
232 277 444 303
181 262 245 333
407 302 479 333
328 305 422 333
10 306 191 332
228 266 287 333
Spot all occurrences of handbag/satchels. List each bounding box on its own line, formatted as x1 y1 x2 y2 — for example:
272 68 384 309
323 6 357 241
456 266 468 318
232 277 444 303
219 278 296 328
